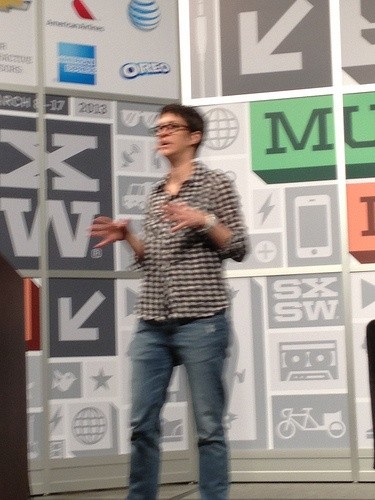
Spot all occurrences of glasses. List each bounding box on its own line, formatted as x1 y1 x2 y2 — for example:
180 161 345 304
149 123 195 134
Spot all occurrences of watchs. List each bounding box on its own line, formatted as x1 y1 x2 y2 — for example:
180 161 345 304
196 210 220 235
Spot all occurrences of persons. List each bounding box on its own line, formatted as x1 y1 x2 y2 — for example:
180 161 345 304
87 101 252 500
